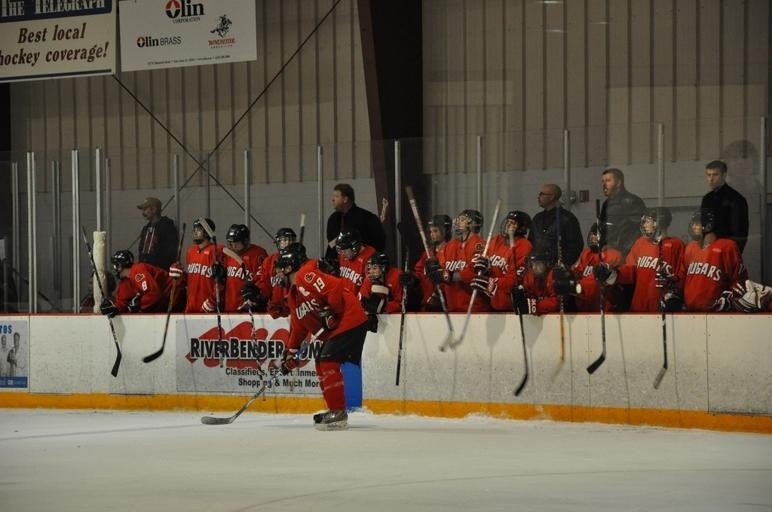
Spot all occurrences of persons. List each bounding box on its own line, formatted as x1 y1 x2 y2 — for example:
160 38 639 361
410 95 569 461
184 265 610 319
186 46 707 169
6 333 27 377
275 252 368 424
0 335 11 376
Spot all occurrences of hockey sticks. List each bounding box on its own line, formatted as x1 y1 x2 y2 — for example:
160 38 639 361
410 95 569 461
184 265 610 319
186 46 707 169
494 228 529 397
142 225 186 363
404 186 453 351
200 217 265 403
79 226 122 378
550 204 566 383
586 201 607 375
651 214 668 390
202 325 325 424
451 199 503 347
395 222 414 386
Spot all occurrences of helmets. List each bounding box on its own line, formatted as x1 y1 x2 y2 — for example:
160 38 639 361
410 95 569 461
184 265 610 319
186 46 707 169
687 210 716 242
427 209 484 244
111 250 133 279
226 224 250 255
273 228 306 275
336 230 361 261
587 222 616 253
365 251 389 284
527 245 556 281
500 211 530 239
639 207 672 238
192 218 215 245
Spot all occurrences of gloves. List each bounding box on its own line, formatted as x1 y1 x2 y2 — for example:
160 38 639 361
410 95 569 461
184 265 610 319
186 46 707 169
712 290 734 312
469 257 499 298
319 306 340 332
99 297 119 318
212 261 226 285
511 285 536 315
425 257 455 287
126 292 142 312
199 295 217 312
400 268 420 289
280 347 300 377
594 262 618 288
656 271 682 312
552 260 580 297
168 261 184 279
240 282 268 305
370 283 394 302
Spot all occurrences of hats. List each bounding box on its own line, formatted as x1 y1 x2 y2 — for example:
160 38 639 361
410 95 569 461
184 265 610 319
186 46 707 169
137 197 161 209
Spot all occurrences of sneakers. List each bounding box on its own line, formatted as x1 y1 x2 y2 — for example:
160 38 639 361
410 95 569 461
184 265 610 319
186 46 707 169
314 408 348 424
